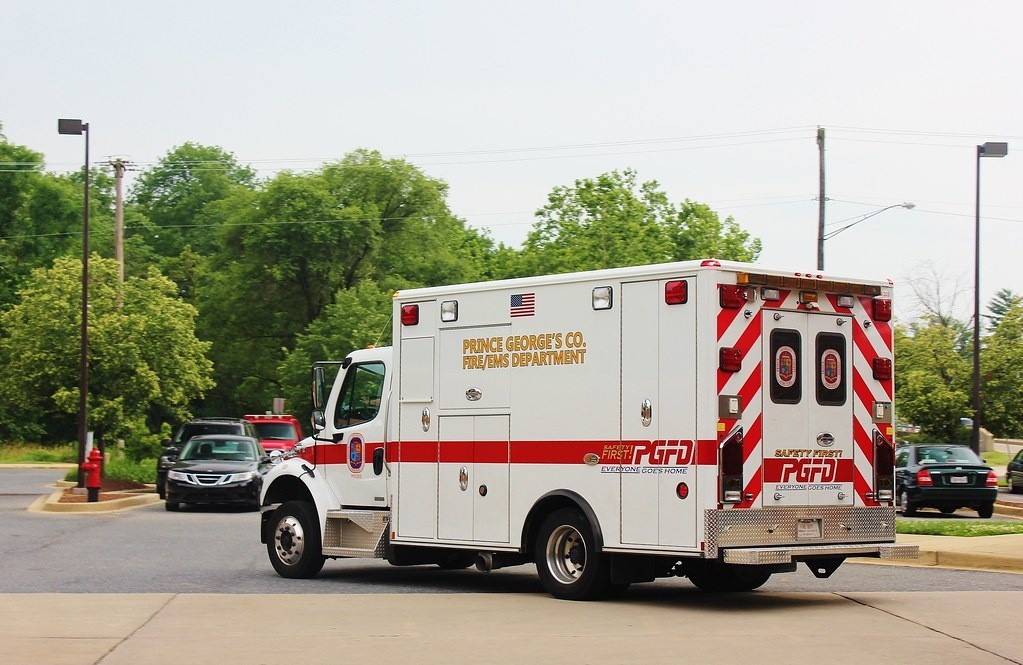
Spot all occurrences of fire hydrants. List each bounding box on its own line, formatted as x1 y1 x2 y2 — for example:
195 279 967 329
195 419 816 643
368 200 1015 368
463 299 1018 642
80 447 103 501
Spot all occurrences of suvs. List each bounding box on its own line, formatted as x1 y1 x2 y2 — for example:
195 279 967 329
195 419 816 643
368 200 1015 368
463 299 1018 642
243 414 304 460
156 416 260 500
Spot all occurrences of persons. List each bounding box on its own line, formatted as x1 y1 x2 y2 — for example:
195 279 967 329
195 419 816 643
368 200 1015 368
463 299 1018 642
359 395 381 406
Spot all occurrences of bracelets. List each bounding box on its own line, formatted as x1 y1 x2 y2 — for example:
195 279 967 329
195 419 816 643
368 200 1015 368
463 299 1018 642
369 400 371 405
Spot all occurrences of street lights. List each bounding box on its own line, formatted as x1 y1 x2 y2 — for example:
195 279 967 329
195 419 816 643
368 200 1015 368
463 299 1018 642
816 200 916 270
970 142 1010 453
57 118 91 488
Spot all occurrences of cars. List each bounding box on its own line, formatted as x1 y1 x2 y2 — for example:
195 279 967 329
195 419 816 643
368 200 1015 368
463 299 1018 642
896 445 998 518
1005 449 1023 494
165 434 273 512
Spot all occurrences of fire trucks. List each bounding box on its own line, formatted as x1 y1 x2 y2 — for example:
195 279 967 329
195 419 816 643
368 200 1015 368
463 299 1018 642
259 259 896 601
